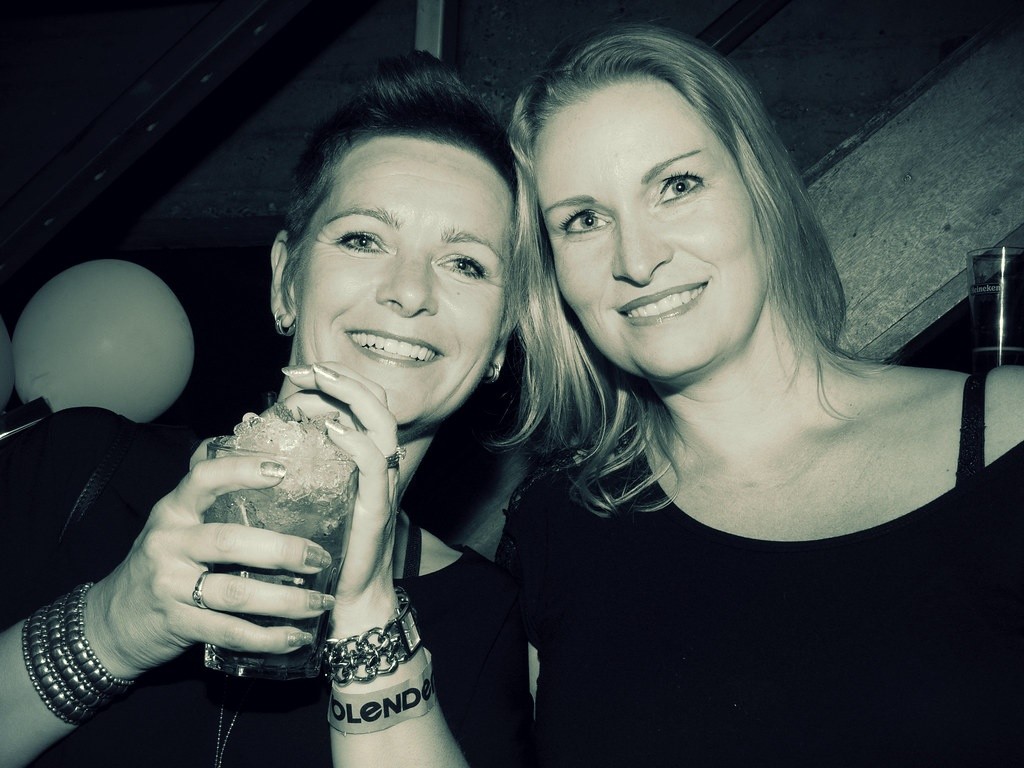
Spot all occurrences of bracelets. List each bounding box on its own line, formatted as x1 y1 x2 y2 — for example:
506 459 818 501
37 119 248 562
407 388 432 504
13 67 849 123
325 648 436 735
21 585 134 725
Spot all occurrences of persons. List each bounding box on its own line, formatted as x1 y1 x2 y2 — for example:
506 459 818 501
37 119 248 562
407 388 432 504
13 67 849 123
493 24 1024 768
0 46 527 768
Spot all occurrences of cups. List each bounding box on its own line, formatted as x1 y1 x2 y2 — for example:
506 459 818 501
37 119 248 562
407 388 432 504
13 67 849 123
968 247 1024 376
202 438 359 680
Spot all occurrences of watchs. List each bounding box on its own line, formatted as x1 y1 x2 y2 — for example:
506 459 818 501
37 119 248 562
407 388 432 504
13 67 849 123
322 582 424 687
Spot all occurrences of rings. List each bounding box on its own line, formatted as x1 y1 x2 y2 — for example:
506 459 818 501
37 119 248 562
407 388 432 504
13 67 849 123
387 445 406 469
192 571 212 609
